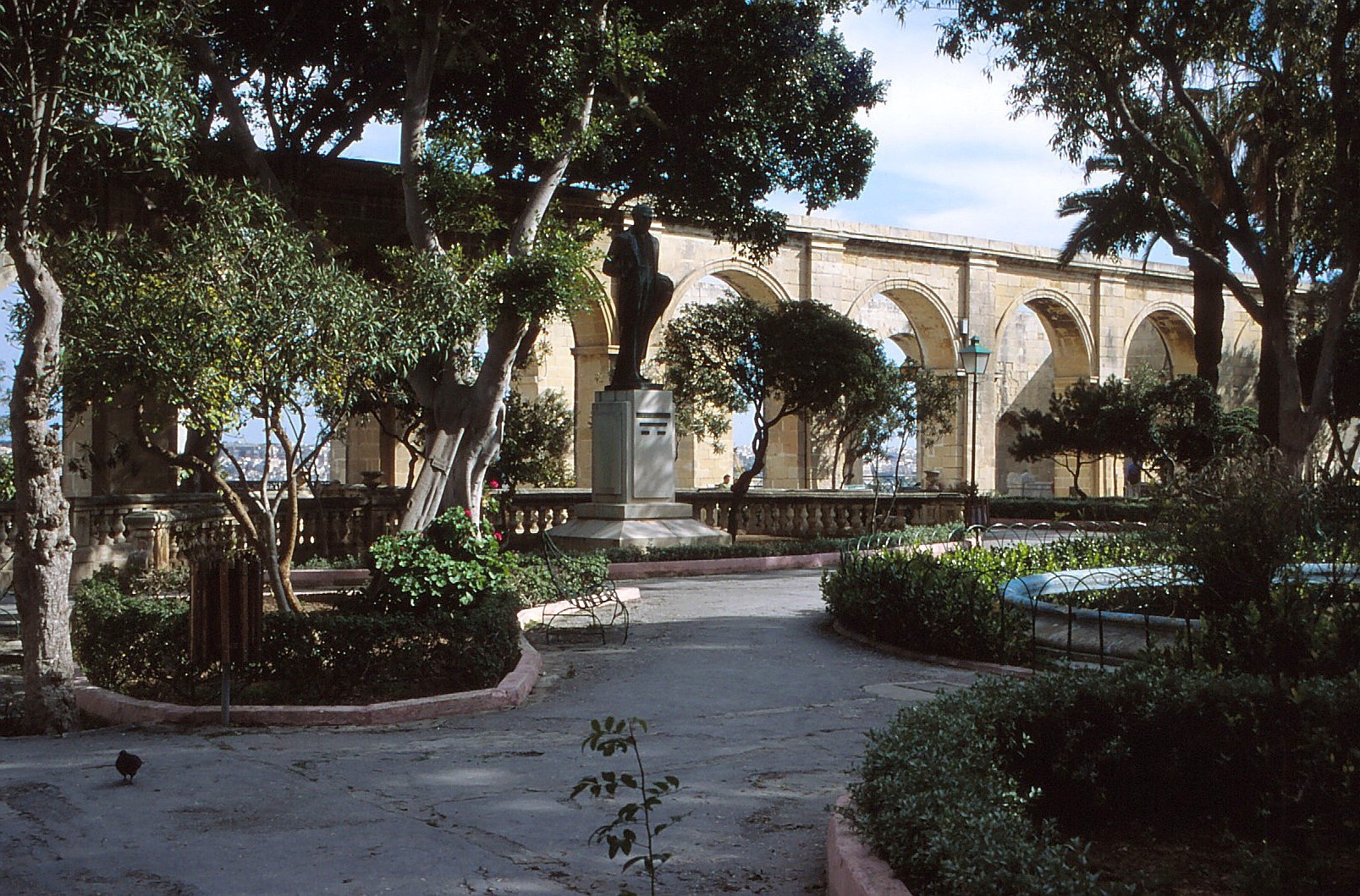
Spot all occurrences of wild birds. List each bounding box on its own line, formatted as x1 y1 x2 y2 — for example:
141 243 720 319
116 750 143 785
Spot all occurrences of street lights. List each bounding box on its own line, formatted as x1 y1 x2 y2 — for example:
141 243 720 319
958 335 994 533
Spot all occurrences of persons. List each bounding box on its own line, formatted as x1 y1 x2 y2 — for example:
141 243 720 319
1125 456 1140 491
718 475 732 489
602 203 660 384
